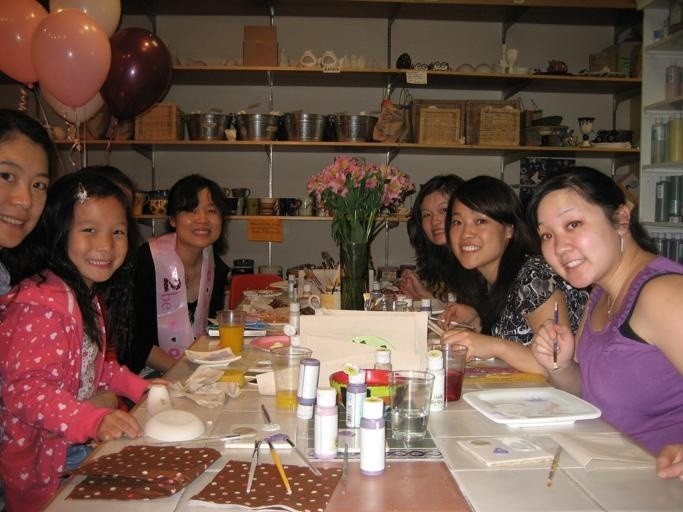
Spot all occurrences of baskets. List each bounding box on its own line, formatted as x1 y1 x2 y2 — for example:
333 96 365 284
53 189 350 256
418 108 461 146
478 107 520 147
465 99 523 145
134 102 184 141
414 98 467 148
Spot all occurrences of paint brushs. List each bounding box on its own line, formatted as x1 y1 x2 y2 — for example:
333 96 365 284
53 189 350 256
391 269 419 286
429 316 475 331
304 266 340 294
546 446 563 487
343 442 349 494
262 405 271 424
247 441 263 493
553 301 561 370
268 440 293 495
148 430 258 447
286 439 322 476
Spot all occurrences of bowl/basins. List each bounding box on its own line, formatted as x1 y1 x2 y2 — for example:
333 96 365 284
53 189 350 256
232 258 254 267
234 113 280 141
147 199 168 217
148 189 170 200
258 265 282 275
331 114 378 143
230 267 254 276
181 112 233 139
282 112 326 141
328 368 409 416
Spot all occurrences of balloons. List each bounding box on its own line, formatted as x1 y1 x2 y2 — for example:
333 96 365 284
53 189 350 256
0 0 49 90
49 0 122 39
29 7 112 112
99 27 173 125
38 81 105 125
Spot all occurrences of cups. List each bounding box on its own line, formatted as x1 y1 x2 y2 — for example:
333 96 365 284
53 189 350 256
277 197 302 216
268 346 313 411
428 343 468 403
245 197 259 216
295 197 313 216
576 116 594 148
260 196 278 216
131 192 150 216
387 369 435 444
216 309 246 353
230 187 250 198
307 292 340 312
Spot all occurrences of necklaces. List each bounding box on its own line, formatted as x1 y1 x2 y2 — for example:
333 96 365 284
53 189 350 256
607 249 641 318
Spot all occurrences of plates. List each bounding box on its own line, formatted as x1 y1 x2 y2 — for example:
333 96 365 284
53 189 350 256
249 335 289 353
184 346 241 369
460 385 602 430
267 280 289 290
141 409 206 443
243 288 282 298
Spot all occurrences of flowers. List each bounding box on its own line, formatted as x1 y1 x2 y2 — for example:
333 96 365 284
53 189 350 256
302 154 421 309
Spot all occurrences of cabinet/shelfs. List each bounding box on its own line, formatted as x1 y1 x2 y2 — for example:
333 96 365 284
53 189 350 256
48 0 645 229
637 2 682 269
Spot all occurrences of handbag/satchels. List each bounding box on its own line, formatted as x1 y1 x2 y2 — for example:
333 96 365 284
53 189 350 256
373 99 414 143
524 109 544 126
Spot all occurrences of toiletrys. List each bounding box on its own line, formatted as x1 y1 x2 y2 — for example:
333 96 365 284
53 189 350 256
663 60 680 104
651 231 683 261
649 116 666 166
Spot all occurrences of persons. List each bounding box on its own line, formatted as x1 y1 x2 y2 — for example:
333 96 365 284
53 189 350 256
79 164 137 369
0 107 59 297
525 165 683 481
0 171 174 512
435 174 592 381
122 173 231 411
399 173 465 300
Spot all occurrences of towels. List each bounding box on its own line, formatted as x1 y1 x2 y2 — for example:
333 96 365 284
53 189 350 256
189 459 345 512
55 445 223 502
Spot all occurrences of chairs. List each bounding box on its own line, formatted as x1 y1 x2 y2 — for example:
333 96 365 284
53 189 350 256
230 274 284 316
297 269 340 292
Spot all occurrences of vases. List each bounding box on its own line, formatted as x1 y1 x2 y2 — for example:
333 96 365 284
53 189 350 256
337 242 370 310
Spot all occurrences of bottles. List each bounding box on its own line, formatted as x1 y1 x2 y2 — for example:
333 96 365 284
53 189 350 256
650 116 665 164
660 115 671 163
672 233 682 263
664 59 680 100
295 358 319 420
668 175 681 225
287 274 295 299
302 276 312 295
370 282 381 302
664 231 674 260
345 370 367 429
373 348 393 370
312 388 339 461
288 302 300 335
403 297 413 312
419 299 432 318
426 350 445 414
359 396 385 477
391 293 406 308
655 231 665 256
649 231 657 244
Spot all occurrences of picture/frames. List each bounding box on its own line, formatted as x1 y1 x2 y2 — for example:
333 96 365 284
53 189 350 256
240 24 281 66
